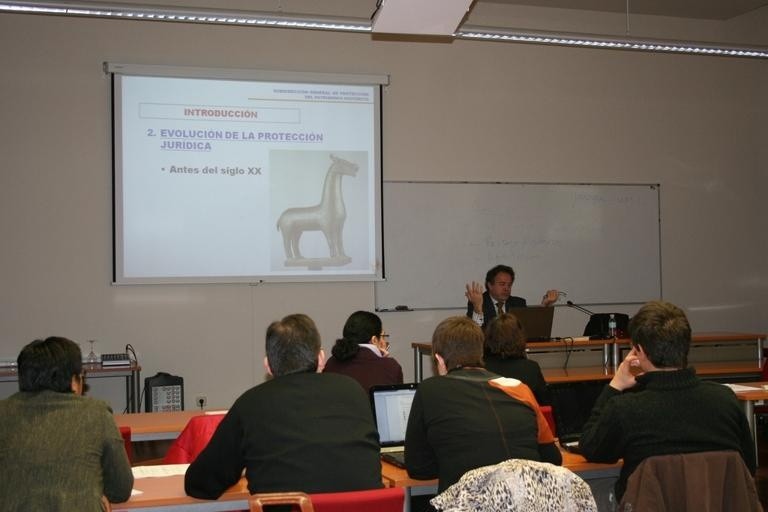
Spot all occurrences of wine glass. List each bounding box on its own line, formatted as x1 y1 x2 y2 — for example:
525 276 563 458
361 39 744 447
85 339 97 364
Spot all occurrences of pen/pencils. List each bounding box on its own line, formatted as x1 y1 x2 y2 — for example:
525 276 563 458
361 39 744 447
381 344 391 355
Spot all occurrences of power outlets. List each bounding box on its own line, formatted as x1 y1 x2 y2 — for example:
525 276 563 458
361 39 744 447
194 394 206 409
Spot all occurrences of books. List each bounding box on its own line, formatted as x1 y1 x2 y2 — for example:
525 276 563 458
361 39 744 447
564 336 589 342
101 353 131 367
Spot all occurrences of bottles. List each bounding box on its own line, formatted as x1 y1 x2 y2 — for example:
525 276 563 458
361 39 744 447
608 314 616 337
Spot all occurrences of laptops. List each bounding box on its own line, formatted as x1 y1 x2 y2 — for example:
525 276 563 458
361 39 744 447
369 383 420 469
508 305 555 342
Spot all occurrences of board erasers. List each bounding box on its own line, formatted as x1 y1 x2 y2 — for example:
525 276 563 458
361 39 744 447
397 307 408 311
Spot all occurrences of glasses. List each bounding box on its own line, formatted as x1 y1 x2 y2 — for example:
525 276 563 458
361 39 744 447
379 335 389 341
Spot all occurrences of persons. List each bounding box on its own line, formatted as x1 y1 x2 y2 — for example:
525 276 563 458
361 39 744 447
0 335 136 512
580 301 761 512
318 311 407 393
464 264 560 345
185 311 389 512
405 316 566 512
478 313 553 406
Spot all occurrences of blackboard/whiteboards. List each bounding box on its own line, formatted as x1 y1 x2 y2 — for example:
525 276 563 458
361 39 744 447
374 181 663 312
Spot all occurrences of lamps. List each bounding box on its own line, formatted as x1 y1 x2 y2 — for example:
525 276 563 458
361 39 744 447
0 1 767 61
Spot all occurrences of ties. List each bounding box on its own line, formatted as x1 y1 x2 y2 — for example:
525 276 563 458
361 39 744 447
495 302 504 316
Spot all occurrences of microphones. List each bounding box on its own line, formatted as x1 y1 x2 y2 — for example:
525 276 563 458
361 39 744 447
567 300 612 340
